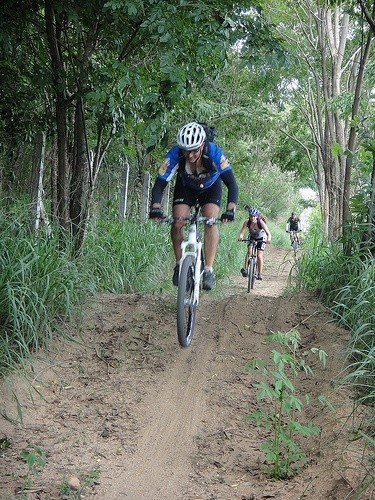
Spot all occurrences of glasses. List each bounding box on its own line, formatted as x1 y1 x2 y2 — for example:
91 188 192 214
182 148 200 154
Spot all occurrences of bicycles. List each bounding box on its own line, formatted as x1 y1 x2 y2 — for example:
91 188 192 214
287 230 302 257
153 199 234 347
237 236 270 293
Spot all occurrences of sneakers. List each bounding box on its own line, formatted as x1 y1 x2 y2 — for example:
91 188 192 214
257 273 263 280
173 265 180 286
241 268 247 278
203 270 213 291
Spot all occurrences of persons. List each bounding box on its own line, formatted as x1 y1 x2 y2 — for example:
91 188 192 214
149 123 239 292
285 212 302 247
238 207 271 280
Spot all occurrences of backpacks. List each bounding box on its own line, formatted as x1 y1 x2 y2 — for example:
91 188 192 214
247 212 267 229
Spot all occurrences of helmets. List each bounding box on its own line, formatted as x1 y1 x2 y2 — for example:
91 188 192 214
176 122 206 151
249 207 259 216
